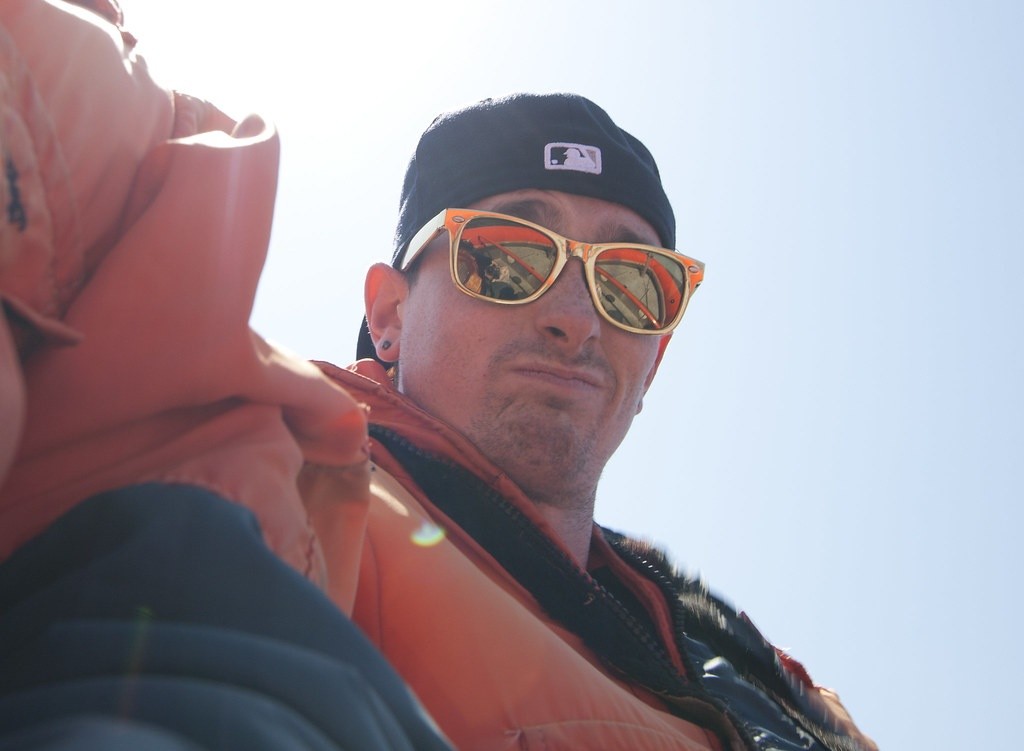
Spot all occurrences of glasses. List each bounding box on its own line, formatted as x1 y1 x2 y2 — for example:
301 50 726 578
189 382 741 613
398 205 709 339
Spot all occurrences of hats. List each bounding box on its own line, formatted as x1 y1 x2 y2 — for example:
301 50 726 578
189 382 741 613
387 92 678 261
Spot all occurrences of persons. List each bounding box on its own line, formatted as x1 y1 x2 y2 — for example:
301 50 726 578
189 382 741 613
0 0 876 751
